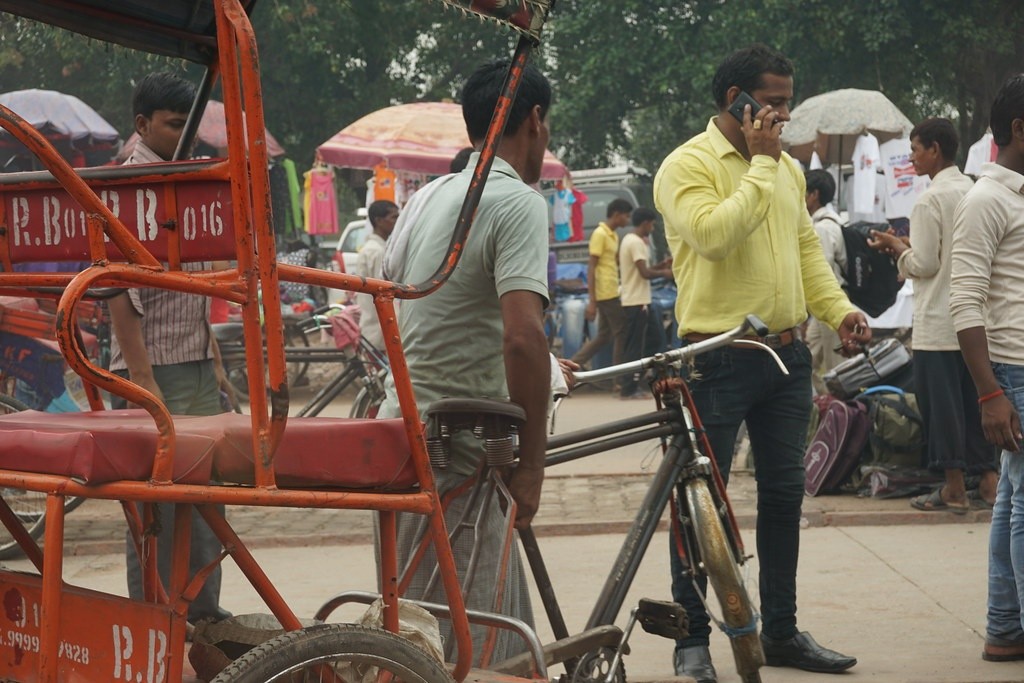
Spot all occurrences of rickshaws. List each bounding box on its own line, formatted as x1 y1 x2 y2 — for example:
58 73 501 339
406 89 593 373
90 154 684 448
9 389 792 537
0 2 791 683
1 303 393 562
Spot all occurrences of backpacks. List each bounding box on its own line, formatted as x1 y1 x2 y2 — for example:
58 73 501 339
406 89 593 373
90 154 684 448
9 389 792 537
818 217 906 319
804 393 926 497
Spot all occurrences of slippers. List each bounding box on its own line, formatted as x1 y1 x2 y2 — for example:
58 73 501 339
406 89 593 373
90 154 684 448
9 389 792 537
983 654 1024 662
967 490 994 509
910 488 969 515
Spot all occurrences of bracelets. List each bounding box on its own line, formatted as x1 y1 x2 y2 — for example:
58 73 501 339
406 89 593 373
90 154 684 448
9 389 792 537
978 389 1004 403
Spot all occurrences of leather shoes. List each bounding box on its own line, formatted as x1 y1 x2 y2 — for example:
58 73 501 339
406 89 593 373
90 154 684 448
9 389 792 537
673 646 717 683
759 631 858 674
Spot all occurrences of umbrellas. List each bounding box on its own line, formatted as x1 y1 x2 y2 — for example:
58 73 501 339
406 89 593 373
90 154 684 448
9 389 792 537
779 88 916 214
124 100 284 156
315 99 566 181
0 89 120 140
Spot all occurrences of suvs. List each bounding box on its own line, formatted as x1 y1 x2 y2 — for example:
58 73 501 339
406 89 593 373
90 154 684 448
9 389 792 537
332 219 370 275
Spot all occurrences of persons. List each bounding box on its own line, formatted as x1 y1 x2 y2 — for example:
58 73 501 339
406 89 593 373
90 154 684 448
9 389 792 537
572 199 676 400
97 72 474 642
376 60 579 669
949 73 1024 661
801 117 1002 513
653 43 873 683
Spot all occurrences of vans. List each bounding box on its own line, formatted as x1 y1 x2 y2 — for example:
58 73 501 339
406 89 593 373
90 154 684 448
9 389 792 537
548 162 674 290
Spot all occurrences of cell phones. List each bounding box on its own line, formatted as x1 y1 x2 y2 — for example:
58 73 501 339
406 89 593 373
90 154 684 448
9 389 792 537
727 91 777 129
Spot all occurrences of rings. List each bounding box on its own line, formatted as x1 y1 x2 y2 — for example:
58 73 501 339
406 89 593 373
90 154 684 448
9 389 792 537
754 119 761 130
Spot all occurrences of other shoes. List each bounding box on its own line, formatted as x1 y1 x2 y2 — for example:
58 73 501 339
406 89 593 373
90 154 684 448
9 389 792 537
615 384 652 400
